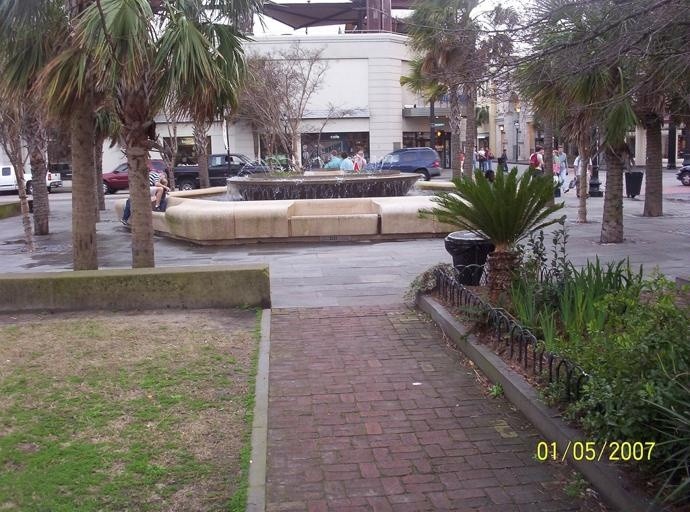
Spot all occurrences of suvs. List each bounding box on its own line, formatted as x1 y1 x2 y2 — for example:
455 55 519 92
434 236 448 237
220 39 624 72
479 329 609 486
102 157 165 196
367 146 443 183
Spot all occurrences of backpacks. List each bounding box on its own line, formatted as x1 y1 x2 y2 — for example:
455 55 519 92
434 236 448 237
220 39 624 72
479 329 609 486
528 153 540 168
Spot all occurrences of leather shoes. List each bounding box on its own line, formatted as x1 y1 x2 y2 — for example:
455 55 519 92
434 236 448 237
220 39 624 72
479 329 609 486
153 204 162 212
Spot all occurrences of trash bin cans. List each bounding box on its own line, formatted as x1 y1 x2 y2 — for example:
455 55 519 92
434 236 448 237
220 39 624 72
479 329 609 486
444 229 495 286
625 172 643 198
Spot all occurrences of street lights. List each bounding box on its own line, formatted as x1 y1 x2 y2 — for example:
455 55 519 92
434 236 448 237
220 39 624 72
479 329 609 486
500 126 505 159
514 122 521 164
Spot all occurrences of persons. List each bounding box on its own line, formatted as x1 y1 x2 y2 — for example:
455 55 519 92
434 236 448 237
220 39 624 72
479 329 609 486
460 146 508 190
324 150 366 171
527 145 593 198
120 169 170 225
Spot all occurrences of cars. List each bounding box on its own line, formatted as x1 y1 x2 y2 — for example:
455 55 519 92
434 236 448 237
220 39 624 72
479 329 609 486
674 165 690 186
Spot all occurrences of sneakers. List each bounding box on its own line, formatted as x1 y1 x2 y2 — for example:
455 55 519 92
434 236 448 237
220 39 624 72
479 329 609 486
120 217 128 225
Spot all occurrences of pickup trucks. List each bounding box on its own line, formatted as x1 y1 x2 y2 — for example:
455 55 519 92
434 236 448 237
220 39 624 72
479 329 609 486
0 165 63 195
166 153 284 193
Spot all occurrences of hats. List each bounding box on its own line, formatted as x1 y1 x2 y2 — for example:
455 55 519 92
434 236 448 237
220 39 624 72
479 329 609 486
330 150 337 156
